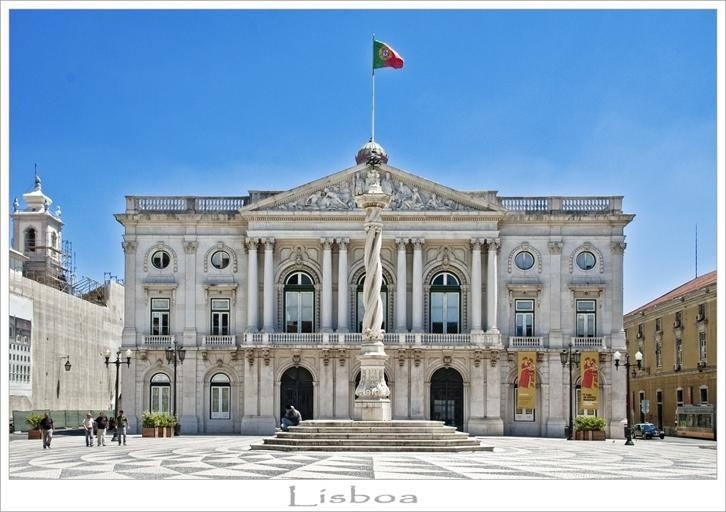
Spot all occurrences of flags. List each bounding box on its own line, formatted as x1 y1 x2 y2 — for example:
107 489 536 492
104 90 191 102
371 40 403 69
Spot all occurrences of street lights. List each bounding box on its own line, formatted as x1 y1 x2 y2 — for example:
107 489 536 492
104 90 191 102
560 341 581 440
165 338 186 435
103 347 132 441
613 347 643 445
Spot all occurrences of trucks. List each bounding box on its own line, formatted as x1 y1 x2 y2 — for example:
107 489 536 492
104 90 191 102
624 420 628 436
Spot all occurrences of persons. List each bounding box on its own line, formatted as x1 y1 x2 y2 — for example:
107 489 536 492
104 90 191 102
382 172 471 210
582 357 598 389
39 412 54 448
115 410 130 445
519 357 534 390
282 404 302 432
83 413 96 447
288 171 365 211
94 411 109 447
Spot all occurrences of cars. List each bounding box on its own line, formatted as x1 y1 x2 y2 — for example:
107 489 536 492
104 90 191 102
631 421 665 440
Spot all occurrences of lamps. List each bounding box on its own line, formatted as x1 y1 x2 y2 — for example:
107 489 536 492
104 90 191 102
59 354 71 371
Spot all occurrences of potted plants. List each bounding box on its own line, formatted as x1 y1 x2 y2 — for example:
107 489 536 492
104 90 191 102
139 410 175 438
23 415 46 440
571 413 607 440
